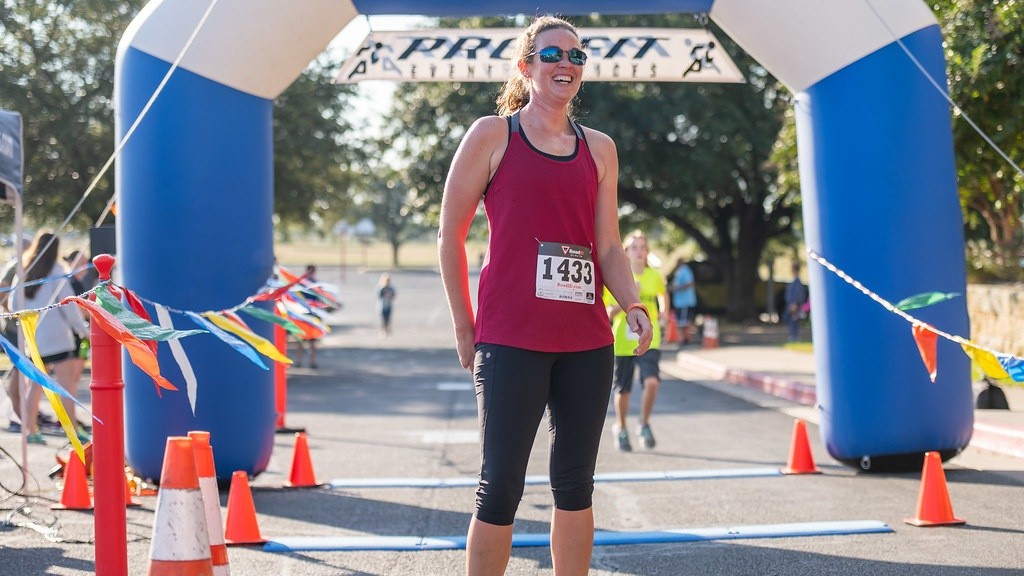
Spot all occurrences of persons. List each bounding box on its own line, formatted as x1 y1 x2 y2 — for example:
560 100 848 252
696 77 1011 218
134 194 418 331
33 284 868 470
438 13 654 576
601 230 668 452
669 258 698 347
62 252 98 377
0 241 52 433
6 232 92 443
294 265 319 368
379 277 395 325
784 263 810 341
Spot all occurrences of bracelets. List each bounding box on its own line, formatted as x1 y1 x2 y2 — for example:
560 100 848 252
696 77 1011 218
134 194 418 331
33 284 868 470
626 303 649 318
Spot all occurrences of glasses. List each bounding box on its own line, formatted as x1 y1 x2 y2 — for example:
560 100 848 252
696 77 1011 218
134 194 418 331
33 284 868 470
523 46 588 65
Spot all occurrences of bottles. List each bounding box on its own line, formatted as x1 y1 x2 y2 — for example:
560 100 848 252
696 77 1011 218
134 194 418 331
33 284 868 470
80 337 89 360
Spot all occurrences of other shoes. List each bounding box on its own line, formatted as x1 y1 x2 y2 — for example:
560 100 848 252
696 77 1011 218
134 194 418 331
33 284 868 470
640 424 655 447
617 431 632 451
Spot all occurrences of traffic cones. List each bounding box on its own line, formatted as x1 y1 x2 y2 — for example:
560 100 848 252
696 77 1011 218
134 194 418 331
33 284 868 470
780 420 823 476
663 310 682 343
699 315 721 350
224 471 268 546
125 473 142 509
281 431 325 489
902 449 968 528
144 436 218 576
48 450 95 511
186 430 233 576
